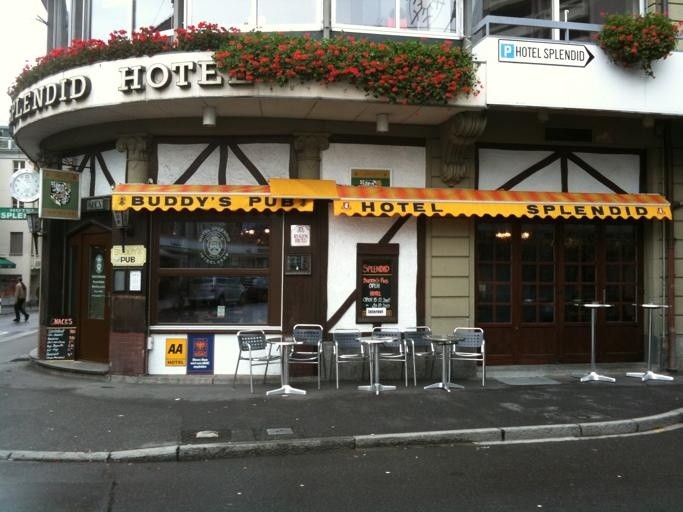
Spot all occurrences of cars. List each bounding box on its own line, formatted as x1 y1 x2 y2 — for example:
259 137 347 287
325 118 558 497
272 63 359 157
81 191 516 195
239 276 268 303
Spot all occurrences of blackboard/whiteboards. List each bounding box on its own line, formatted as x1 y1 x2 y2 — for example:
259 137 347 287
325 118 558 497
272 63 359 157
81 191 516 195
46 317 78 360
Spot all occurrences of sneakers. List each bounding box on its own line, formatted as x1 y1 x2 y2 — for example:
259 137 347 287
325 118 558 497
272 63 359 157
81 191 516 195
24 314 30 321
12 318 20 323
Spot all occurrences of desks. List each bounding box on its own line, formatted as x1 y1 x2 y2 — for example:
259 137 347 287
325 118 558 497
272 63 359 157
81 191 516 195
425 332 465 394
267 338 306 397
574 299 617 382
626 303 674 381
355 334 394 395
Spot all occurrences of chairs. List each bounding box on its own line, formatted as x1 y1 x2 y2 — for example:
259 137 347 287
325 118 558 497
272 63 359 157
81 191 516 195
448 327 487 388
286 324 325 390
374 325 408 387
236 330 285 399
334 330 370 388
407 326 439 386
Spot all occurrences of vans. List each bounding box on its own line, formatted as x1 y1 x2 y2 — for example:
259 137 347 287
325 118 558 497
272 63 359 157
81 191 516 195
186 275 248 308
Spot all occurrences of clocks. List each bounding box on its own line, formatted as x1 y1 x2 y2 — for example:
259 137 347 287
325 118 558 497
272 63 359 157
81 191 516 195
8 167 39 204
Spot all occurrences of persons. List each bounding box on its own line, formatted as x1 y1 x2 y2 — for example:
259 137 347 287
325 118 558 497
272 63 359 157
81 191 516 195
12 277 29 321
